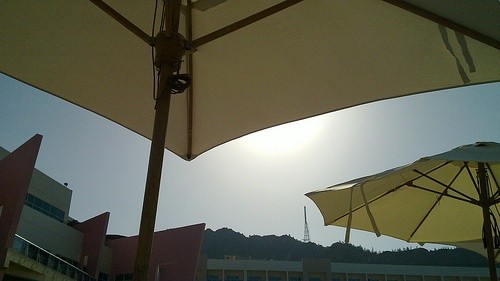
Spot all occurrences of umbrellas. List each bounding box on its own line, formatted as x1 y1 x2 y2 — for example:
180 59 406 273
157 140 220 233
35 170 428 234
0 0 500 281
304 139 499 281
